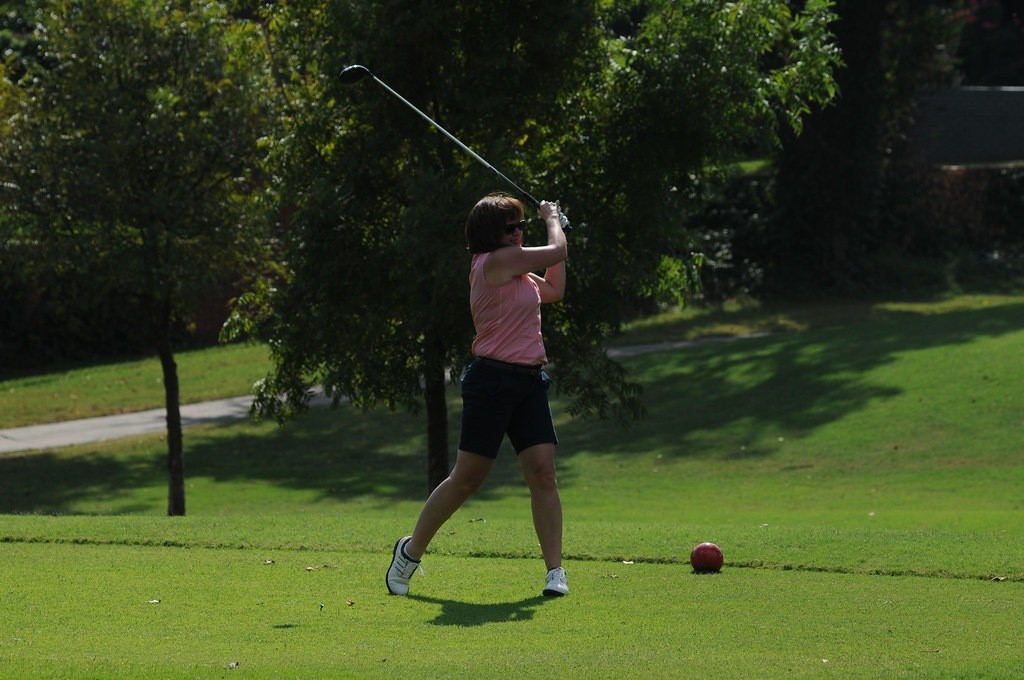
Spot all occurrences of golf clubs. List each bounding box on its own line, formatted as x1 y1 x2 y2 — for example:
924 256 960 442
338 65 575 235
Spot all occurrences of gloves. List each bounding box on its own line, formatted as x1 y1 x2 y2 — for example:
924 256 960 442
559 212 570 234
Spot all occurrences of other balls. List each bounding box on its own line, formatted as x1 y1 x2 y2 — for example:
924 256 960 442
689 542 724 575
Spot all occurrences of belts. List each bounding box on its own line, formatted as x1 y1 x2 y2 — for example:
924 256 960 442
475 357 541 376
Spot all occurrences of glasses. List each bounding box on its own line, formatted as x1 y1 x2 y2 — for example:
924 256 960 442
504 220 526 234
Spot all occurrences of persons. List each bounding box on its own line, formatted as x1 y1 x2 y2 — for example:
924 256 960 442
386 192 568 596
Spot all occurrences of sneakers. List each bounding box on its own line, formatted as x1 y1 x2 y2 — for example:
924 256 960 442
543 566 568 596
386 536 425 596
